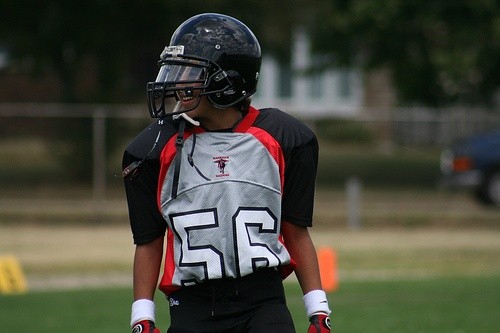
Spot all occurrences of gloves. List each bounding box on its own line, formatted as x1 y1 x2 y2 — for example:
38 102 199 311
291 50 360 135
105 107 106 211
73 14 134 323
307 312 331 333
133 320 161 333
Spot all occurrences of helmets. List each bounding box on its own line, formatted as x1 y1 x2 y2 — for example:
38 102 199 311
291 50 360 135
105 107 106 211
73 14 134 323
161 13 262 110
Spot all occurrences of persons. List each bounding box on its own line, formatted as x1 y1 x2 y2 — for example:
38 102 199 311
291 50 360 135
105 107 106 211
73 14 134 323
121 12 333 333
